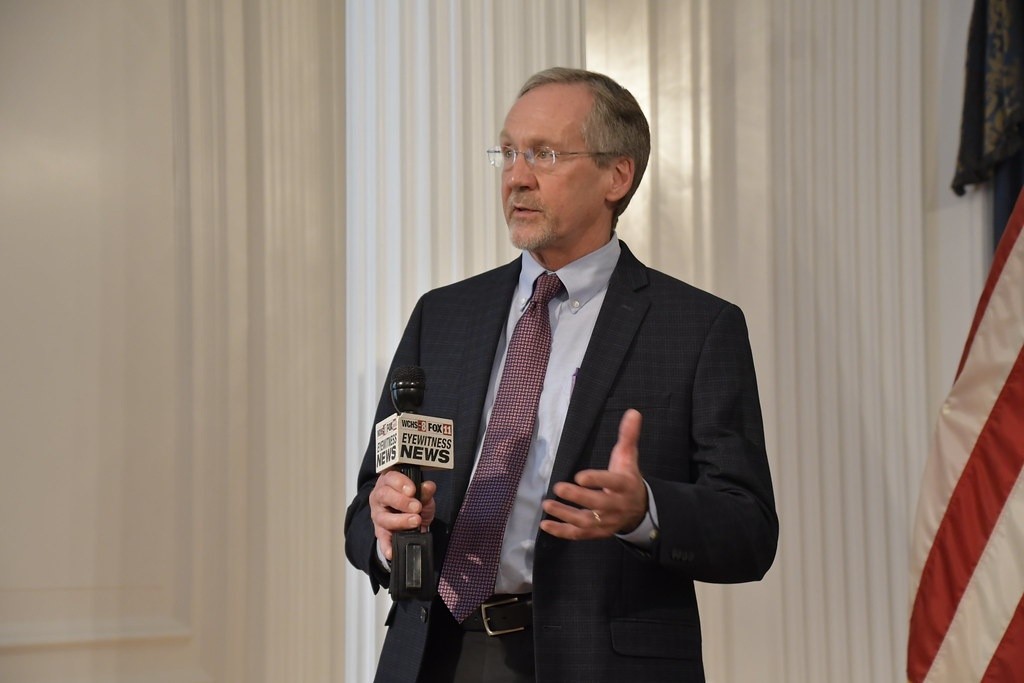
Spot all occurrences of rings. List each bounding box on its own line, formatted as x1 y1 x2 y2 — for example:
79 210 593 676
592 509 602 525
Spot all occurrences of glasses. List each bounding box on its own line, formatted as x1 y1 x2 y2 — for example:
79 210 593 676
486 144 624 174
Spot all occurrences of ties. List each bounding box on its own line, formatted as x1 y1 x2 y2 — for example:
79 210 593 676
436 272 565 623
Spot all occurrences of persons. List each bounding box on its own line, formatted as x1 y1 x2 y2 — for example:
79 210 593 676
344 66 780 683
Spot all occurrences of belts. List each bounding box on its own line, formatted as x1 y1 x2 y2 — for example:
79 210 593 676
452 592 533 636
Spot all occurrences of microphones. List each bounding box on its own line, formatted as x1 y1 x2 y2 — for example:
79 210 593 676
374 363 454 604
